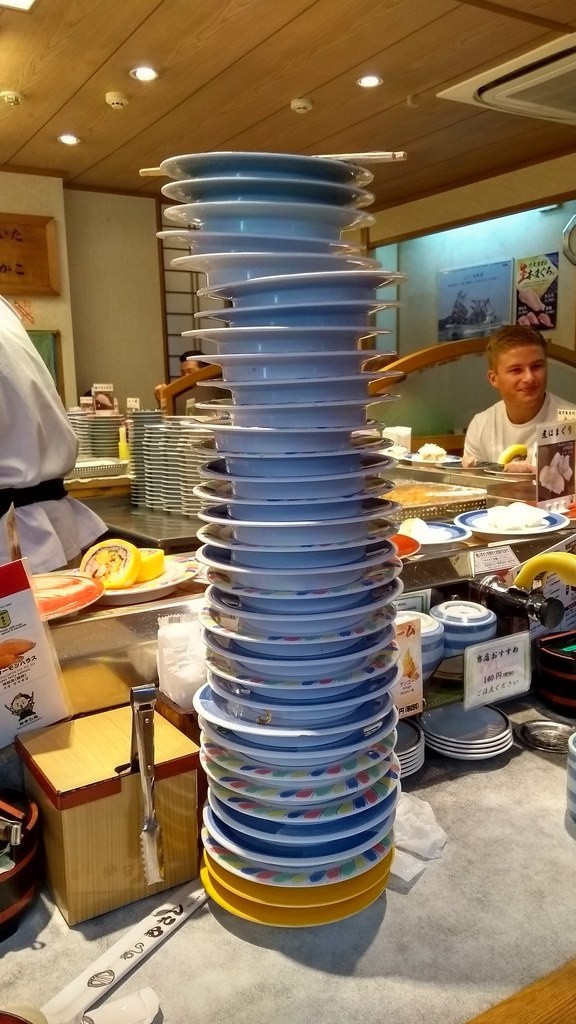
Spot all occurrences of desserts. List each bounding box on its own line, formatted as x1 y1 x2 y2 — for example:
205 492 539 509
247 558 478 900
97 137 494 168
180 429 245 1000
417 443 446 461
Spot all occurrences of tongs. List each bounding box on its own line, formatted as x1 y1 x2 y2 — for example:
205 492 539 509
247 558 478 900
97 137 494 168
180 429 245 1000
0 816 22 846
128 681 167 886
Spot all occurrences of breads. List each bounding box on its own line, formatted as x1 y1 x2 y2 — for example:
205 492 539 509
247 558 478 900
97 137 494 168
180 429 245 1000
78 538 165 589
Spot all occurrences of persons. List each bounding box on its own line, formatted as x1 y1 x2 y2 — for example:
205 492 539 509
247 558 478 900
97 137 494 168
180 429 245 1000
152 349 210 403
0 294 110 574
462 325 576 466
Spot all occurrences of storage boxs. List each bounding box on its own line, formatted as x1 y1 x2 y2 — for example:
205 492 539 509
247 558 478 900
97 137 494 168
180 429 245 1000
14 698 201 919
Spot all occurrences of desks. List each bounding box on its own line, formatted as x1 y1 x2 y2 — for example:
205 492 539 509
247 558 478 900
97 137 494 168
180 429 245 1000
67 490 209 555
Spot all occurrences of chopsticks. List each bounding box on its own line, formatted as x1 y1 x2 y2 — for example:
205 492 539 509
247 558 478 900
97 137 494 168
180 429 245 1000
139 151 409 177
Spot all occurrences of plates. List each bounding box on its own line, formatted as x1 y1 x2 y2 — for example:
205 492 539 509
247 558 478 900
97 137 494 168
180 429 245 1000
416 704 514 760
148 146 415 931
516 719 576 754
65 555 201 606
389 718 425 780
404 452 536 478
388 508 570 559
28 574 105 622
65 410 212 519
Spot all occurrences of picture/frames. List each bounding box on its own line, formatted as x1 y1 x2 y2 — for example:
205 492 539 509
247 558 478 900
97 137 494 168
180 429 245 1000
434 257 515 347
